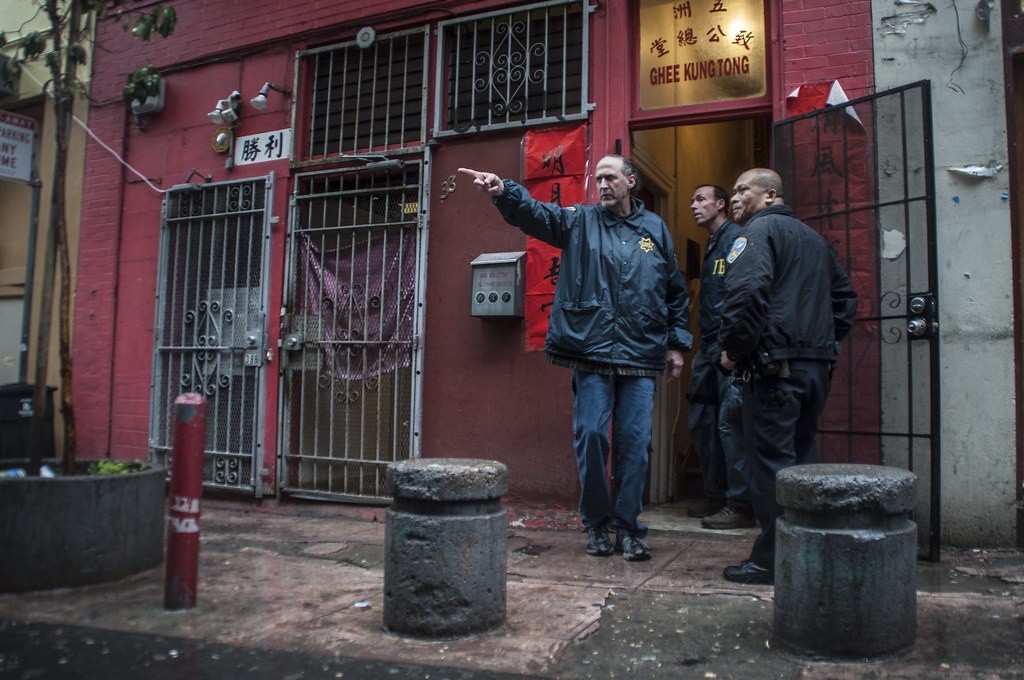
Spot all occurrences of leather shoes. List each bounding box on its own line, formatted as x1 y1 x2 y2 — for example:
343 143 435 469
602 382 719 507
724 558 774 585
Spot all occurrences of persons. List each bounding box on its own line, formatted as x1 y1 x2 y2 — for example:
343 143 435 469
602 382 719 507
718 168 858 585
458 154 692 561
691 185 757 531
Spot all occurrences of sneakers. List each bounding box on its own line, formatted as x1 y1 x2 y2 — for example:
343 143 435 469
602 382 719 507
583 526 617 555
702 503 757 529
614 532 652 561
687 497 726 517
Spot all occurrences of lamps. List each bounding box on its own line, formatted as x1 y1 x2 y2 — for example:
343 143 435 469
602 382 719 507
207 91 242 168
251 81 291 112
